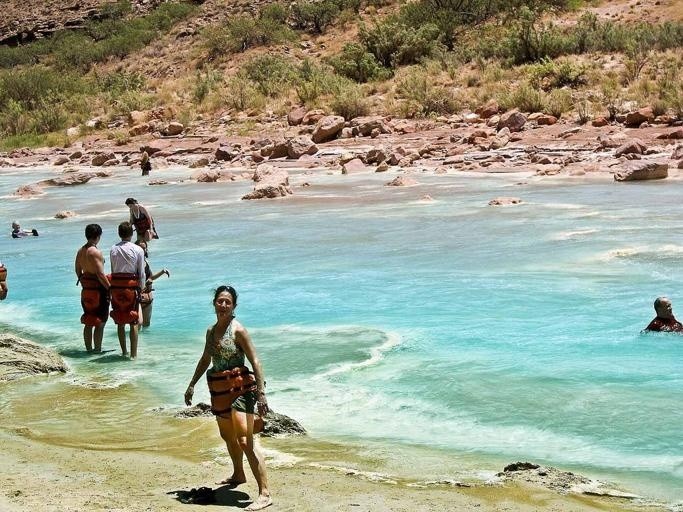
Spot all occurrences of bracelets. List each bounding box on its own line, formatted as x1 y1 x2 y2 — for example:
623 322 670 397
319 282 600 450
191 381 196 385
257 392 265 397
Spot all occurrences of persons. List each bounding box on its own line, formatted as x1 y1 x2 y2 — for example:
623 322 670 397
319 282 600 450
75 224 112 354
134 238 170 333
125 198 159 241
639 297 683 334
12 220 38 238
184 286 273 511
109 222 146 361
0 261 8 300
139 146 152 176
352 127 359 143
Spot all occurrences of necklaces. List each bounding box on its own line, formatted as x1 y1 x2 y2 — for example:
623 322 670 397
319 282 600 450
132 204 140 219
86 242 105 264
